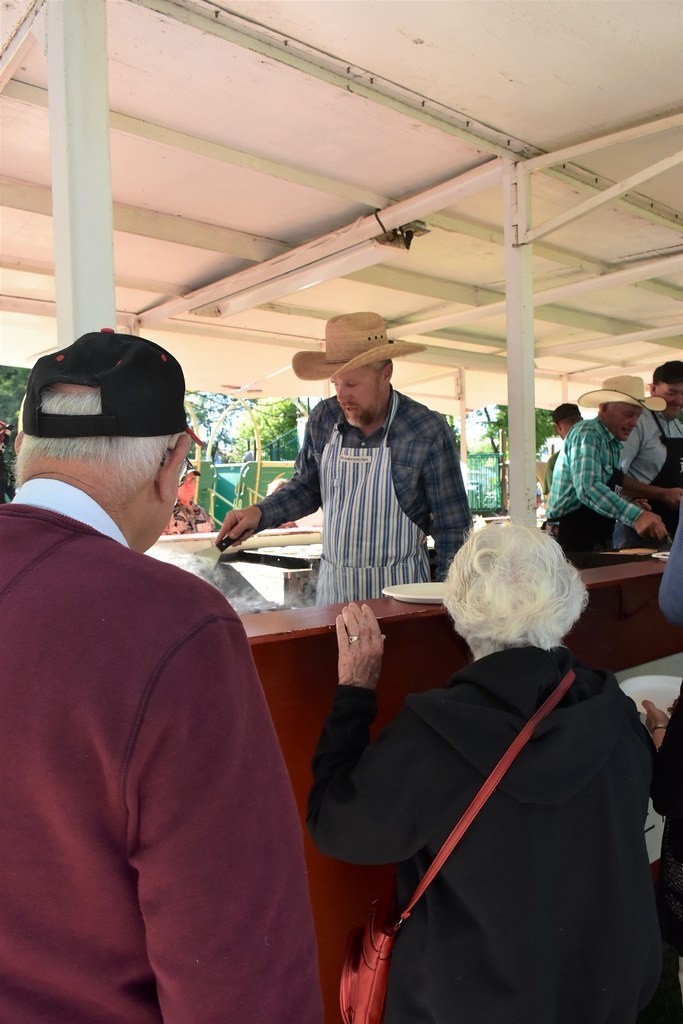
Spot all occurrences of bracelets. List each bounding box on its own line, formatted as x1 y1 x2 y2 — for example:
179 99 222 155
652 726 667 733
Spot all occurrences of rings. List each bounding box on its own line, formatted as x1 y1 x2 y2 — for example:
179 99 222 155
348 636 360 644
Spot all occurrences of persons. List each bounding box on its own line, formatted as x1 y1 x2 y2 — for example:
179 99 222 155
658 495 683 626
306 513 662 1024
635 680 683 1024
0 326 324 1024
544 400 586 497
614 360 683 552
161 463 217 535
544 373 668 571
215 310 473 605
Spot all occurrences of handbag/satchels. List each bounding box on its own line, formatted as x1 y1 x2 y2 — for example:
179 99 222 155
340 892 394 1024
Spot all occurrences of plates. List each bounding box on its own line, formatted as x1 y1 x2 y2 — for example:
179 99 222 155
618 675 683 719
652 552 670 561
382 583 445 603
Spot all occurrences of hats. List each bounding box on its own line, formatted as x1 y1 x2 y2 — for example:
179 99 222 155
293 311 427 381
578 376 668 412
22 329 204 447
552 404 581 422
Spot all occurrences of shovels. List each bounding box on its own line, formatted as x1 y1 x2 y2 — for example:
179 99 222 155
175 529 249 579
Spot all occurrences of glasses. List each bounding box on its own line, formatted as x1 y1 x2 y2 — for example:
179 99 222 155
161 449 201 486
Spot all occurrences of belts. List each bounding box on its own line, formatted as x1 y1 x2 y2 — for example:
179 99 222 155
545 524 559 537
